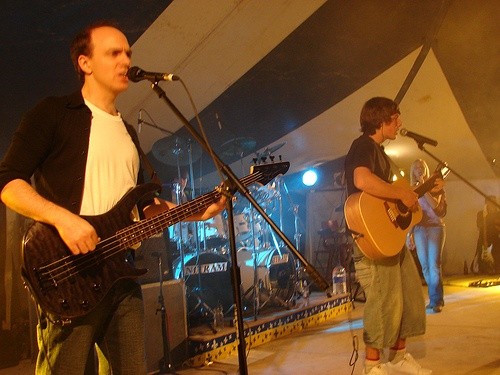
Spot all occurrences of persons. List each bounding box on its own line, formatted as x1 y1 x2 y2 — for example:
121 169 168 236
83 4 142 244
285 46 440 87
0 22 235 375
342 95 444 375
476 195 500 275
404 158 447 313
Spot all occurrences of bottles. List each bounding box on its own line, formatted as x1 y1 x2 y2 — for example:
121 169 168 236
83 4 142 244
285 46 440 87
331 262 346 295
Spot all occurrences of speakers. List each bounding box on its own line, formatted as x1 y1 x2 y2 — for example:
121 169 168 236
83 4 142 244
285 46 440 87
135 227 173 286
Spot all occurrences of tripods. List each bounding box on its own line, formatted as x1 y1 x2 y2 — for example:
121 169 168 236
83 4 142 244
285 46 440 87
141 121 290 334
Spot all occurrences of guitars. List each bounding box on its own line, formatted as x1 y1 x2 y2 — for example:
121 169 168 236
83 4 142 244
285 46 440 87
20 155 290 324
477 203 496 274
344 162 450 261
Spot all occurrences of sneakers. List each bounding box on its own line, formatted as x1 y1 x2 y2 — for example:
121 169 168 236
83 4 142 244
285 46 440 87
362 363 396 375
388 353 433 375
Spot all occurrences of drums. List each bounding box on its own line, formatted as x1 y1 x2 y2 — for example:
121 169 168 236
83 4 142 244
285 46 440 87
224 214 250 239
240 208 263 244
168 221 196 253
235 247 296 305
195 211 228 249
173 253 239 319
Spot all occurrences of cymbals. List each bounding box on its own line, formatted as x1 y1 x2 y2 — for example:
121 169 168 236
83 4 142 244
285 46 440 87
248 142 286 166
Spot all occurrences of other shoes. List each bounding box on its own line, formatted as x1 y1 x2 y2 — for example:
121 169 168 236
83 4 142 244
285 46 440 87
426 300 444 313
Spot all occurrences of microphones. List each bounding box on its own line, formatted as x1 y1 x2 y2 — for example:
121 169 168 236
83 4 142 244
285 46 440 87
137 110 142 135
400 128 438 147
127 66 178 82
